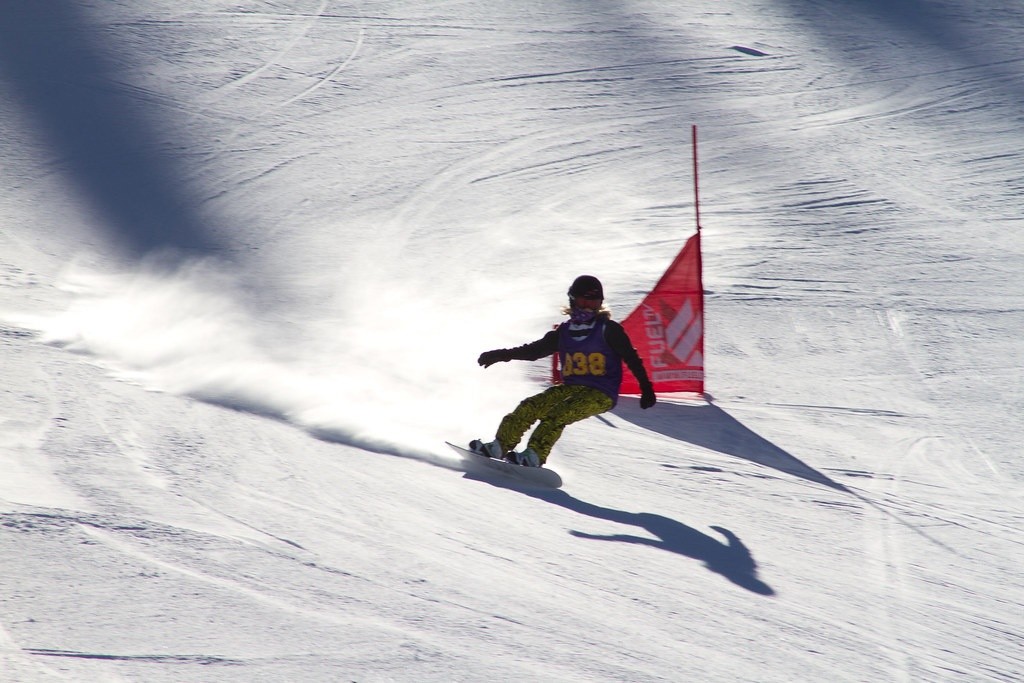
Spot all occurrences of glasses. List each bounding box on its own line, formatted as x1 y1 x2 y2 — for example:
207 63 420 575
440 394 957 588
576 299 601 308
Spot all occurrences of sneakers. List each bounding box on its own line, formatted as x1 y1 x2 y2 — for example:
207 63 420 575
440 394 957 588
469 438 509 462
505 448 541 468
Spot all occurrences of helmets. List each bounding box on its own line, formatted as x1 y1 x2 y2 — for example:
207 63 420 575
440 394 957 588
568 275 604 301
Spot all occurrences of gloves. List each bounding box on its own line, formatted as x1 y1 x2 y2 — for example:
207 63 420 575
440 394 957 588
639 382 657 409
477 348 511 369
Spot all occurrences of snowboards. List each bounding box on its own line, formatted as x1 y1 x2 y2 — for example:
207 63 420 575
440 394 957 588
444 440 563 489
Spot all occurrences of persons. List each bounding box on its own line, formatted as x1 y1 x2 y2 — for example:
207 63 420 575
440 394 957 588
469 276 657 467
